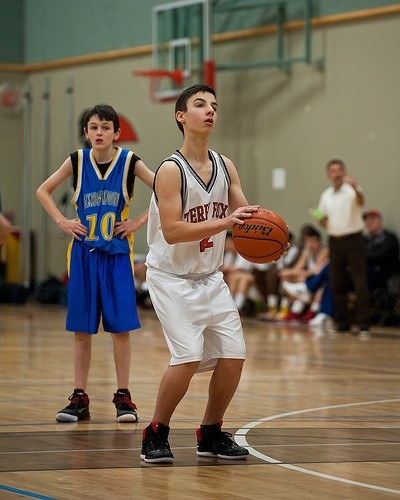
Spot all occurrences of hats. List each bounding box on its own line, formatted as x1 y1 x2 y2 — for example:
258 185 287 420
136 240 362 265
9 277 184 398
362 212 381 220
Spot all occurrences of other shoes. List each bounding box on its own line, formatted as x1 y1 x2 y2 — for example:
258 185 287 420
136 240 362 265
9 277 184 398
259 306 333 327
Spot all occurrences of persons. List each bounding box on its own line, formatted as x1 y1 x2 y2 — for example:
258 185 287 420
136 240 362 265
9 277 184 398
36 103 156 422
138 83 291 464
220 209 400 343
313 159 376 343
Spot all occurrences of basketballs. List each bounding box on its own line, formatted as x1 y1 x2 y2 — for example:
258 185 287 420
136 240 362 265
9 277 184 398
232 208 288 264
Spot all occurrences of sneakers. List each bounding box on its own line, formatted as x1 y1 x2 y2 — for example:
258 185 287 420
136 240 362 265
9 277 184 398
195 420 250 460
112 386 138 423
56 389 91 422
140 420 176 462
